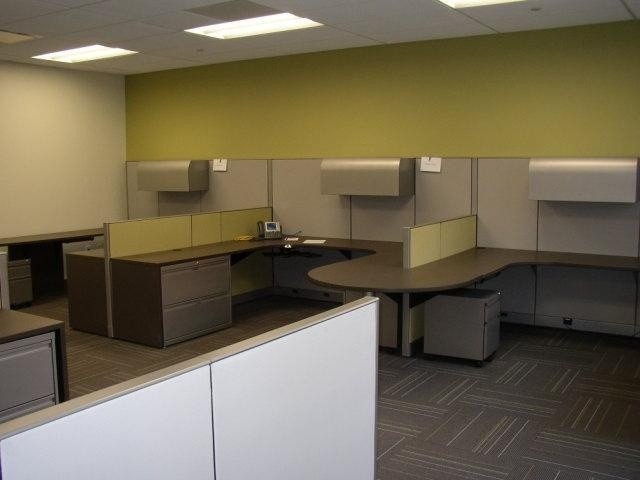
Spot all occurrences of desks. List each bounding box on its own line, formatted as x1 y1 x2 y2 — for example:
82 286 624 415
406 245 640 307
0 228 105 247
110 235 407 349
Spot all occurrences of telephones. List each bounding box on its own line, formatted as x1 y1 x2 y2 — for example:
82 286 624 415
258 221 282 239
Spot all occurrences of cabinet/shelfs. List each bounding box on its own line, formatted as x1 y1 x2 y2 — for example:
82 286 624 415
65 249 109 340
7 258 34 306
0 307 71 423
342 289 402 354
422 286 505 368
